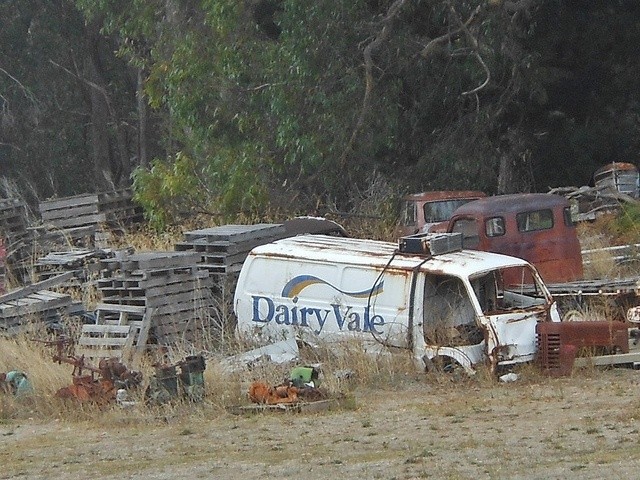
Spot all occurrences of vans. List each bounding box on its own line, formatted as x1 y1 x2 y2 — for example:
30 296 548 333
397 190 488 237
226 232 566 379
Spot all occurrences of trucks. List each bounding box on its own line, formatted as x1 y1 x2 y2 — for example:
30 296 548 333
447 193 640 357
